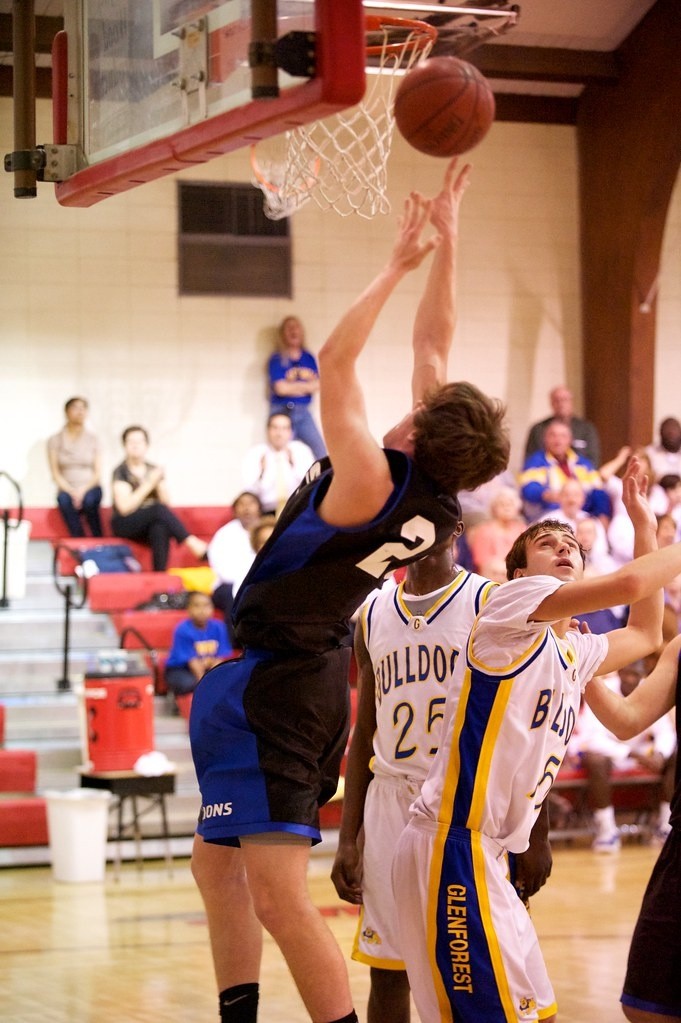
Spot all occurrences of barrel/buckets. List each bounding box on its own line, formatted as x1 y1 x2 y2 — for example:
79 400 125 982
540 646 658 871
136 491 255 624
82 650 157 775
41 788 113 883
0 518 31 601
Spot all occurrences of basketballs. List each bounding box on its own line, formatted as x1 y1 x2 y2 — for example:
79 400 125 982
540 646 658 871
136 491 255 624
393 55 494 157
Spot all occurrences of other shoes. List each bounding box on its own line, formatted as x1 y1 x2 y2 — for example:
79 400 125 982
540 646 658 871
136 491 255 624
653 825 674 843
591 829 620 855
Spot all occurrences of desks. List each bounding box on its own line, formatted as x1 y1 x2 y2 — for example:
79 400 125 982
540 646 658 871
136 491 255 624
78 766 178 879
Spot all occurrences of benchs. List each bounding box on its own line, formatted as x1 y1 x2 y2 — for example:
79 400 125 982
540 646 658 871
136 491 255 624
0 506 681 852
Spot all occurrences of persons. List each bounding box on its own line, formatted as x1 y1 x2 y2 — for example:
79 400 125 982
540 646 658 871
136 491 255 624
111 426 209 572
188 161 681 1023
165 590 229 713
266 316 326 459
243 416 315 514
47 399 104 540
